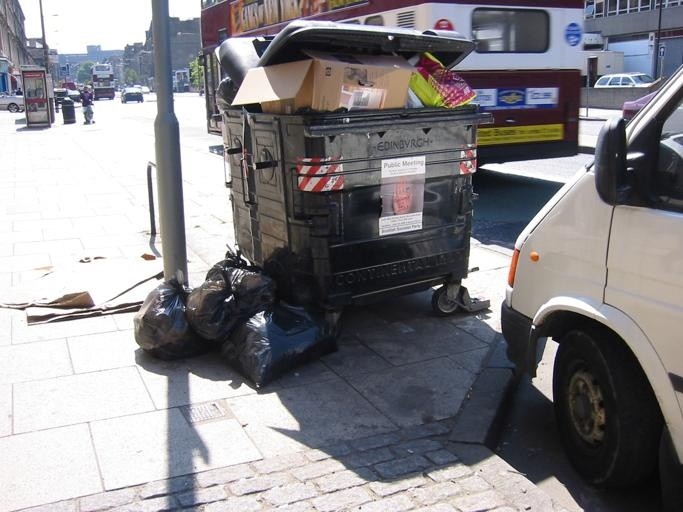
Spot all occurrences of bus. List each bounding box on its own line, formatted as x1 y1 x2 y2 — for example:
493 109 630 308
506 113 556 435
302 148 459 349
194 0 585 176
89 61 116 101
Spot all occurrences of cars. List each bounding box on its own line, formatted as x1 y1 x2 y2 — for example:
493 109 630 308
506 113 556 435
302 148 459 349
120 87 144 105
0 91 26 113
66 89 82 102
133 84 150 95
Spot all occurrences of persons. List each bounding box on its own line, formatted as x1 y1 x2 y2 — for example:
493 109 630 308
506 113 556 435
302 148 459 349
79 86 96 125
16 88 23 95
392 176 413 214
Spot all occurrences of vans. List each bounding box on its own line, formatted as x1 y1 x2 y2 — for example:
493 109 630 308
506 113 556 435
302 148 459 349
594 71 656 88
498 60 683 494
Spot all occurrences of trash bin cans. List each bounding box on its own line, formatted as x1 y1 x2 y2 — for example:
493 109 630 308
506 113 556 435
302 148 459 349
212 21 494 341
61 96 76 124
